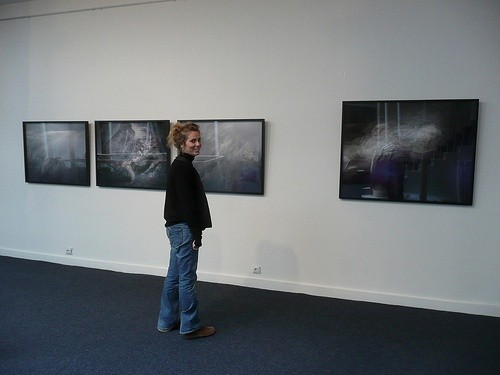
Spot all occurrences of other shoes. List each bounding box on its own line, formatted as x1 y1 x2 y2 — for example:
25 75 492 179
183 327 216 340
158 319 180 333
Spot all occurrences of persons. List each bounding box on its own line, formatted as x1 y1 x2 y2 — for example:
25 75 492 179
156 121 216 339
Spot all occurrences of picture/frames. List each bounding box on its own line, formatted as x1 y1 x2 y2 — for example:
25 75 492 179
177 118 266 195
23 120 91 187
96 118 171 191
339 98 480 207
